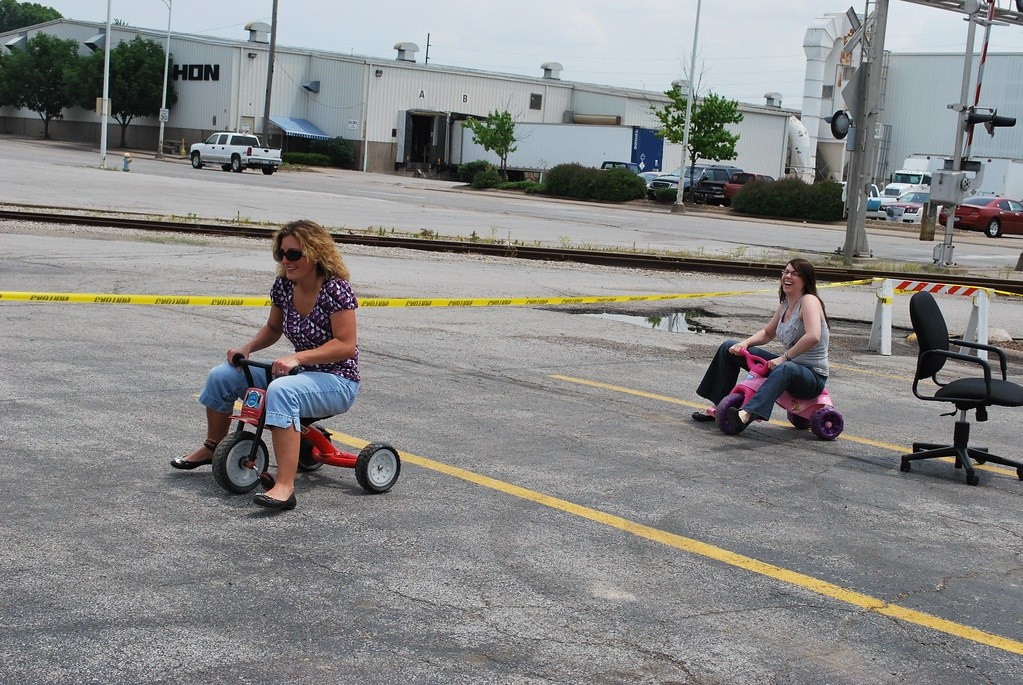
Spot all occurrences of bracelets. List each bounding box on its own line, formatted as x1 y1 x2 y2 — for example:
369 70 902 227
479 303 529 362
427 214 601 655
783 351 791 361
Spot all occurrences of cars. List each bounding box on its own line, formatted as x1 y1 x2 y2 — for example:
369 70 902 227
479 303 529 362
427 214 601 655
938 196 1023 238
636 171 668 192
878 190 931 223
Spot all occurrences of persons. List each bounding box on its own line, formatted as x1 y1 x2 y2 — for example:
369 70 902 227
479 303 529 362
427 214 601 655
692 258 830 432
170 219 362 510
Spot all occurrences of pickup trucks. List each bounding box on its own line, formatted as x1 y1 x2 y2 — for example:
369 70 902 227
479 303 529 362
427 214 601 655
190 132 283 176
601 161 641 177
695 179 727 205
867 184 897 205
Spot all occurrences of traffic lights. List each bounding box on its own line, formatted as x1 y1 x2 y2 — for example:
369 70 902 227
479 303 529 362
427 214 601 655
823 109 850 140
985 108 1017 136
967 108 993 130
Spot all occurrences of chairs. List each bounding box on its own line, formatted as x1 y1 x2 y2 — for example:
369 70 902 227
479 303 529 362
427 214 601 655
891 290 1023 485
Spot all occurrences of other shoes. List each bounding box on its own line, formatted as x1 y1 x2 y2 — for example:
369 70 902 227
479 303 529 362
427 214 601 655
253 493 296 509
692 412 715 422
726 407 745 432
170 454 212 470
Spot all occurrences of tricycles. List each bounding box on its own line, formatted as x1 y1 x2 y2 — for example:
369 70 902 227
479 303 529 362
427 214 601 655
705 348 846 441
203 353 401 495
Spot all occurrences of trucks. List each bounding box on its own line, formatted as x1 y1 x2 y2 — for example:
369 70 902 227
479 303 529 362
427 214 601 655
879 170 932 201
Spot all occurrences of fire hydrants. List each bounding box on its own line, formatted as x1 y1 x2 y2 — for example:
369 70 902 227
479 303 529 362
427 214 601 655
122 152 132 171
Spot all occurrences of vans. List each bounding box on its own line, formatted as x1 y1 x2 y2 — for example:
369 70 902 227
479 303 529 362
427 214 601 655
722 172 775 204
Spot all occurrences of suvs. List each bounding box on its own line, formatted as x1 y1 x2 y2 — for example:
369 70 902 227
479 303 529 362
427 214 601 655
648 163 744 204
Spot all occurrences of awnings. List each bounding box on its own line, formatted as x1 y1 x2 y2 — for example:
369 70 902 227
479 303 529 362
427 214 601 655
268 116 330 141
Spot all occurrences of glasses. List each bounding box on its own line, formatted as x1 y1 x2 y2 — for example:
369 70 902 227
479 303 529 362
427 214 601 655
276 248 307 261
782 269 801 277
